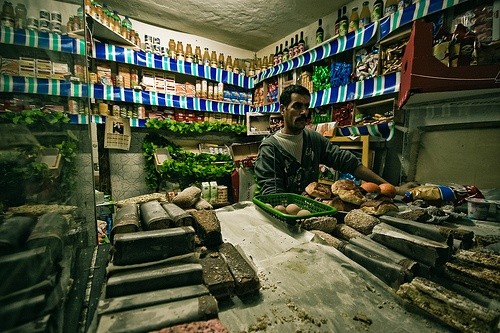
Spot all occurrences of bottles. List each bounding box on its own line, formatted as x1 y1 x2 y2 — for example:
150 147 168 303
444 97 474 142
195 77 224 101
233 31 305 78
85 0 141 49
348 6 359 34
339 5 349 33
1 1 14 27
14 3 27 29
316 18 324 45
168 39 232 72
372 0 423 22
67 4 83 32
359 1 371 28
92 99 145 119
335 9 342 35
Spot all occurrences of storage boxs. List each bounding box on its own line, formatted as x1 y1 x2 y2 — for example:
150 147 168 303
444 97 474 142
355 98 404 124
246 112 269 135
229 141 260 162
330 101 363 127
153 147 171 171
398 18 500 108
199 143 233 164
36 148 64 177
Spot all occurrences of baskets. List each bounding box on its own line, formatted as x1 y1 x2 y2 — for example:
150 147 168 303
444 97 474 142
253 193 338 226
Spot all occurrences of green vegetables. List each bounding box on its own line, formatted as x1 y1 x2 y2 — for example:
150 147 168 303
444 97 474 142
143 118 247 192
0 108 78 195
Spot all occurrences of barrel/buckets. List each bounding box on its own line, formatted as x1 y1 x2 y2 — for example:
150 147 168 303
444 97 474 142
145 35 168 57
28 9 62 34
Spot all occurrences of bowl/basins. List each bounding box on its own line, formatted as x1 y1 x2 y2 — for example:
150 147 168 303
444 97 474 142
494 200 500 222
465 197 496 220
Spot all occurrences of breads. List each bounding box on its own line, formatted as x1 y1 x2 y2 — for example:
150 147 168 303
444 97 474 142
303 180 400 215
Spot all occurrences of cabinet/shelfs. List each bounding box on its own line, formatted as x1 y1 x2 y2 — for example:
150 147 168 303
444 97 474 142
86 0 466 142
0 25 90 125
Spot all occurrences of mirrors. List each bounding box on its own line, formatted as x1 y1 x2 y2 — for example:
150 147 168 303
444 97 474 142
0 0 99 333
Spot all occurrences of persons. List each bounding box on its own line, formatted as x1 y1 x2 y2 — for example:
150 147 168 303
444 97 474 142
254 85 421 196
113 122 123 134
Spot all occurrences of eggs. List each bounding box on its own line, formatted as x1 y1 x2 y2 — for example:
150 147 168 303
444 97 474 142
265 204 312 215
